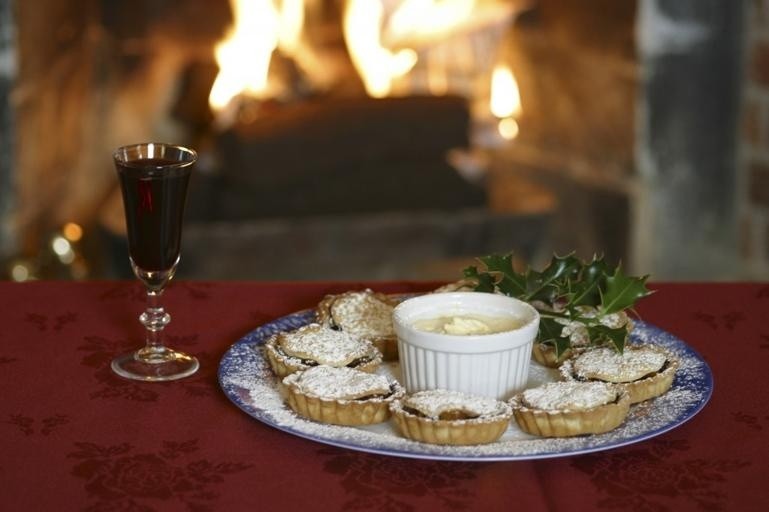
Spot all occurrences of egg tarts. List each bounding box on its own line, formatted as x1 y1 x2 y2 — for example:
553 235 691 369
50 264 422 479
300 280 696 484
263 271 678 446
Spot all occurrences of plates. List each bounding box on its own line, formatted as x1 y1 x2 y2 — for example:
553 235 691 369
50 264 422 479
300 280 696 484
217 292 716 463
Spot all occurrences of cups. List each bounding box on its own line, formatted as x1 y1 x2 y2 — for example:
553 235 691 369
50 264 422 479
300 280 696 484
391 290 541 403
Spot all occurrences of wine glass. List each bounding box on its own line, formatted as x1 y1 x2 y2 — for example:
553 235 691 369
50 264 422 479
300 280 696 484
111 140 201 382
